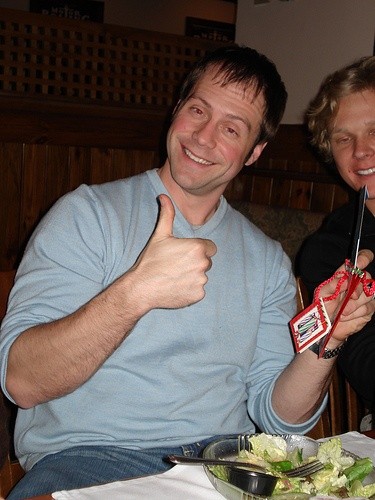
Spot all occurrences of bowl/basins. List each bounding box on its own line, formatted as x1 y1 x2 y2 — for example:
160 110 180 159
202 435 375 500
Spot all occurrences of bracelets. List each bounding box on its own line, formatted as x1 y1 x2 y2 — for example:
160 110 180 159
307 341 345 360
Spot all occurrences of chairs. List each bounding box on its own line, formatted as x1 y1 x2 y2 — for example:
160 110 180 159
228 200 363 442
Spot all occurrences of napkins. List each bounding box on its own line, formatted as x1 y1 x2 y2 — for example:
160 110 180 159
48 428 375 500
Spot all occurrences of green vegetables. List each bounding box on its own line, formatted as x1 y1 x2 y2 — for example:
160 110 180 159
207 430 375 500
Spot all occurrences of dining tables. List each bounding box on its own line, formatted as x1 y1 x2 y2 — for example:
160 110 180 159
15 429 375 500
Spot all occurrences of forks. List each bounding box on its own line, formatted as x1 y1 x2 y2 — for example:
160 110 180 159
162 454 325 479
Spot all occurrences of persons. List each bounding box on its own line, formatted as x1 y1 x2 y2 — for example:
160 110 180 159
0 41 375 500
293 54 374 432
